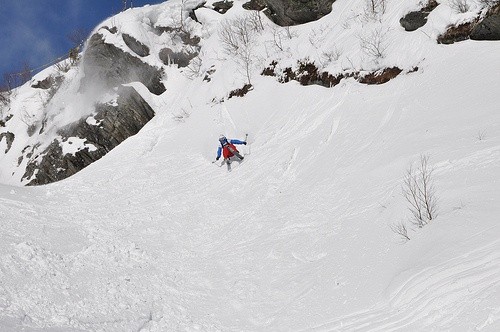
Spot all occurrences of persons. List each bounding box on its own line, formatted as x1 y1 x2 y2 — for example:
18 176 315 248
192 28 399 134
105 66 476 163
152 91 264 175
216 134 247 164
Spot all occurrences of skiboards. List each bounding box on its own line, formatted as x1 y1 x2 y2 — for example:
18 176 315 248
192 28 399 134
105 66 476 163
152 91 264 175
227 159 246 171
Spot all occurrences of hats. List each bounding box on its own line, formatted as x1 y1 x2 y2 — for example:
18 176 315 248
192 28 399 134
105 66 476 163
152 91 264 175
218 134 226 141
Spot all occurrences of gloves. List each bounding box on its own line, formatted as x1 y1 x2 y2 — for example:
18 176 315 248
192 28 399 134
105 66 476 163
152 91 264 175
216 157 219 160
243 142 246 145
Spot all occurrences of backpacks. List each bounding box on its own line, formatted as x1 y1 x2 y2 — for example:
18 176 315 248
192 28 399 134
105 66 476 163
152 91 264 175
222 142 237 159
